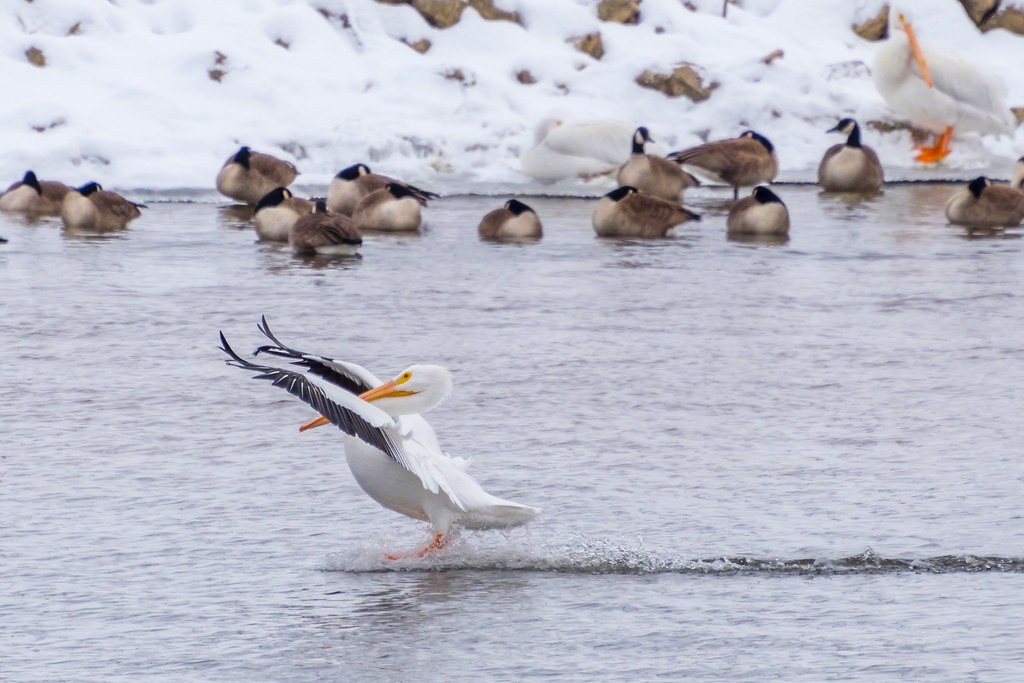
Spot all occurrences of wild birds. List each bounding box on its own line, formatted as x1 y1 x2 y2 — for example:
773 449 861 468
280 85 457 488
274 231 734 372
518 116 674 179
818 118 885 193
945 153 1024 230
218 314 539 562
478 199 544 239
216 145 443 257
872 2 1019 165
593 127 791 238
0 171 149 232
